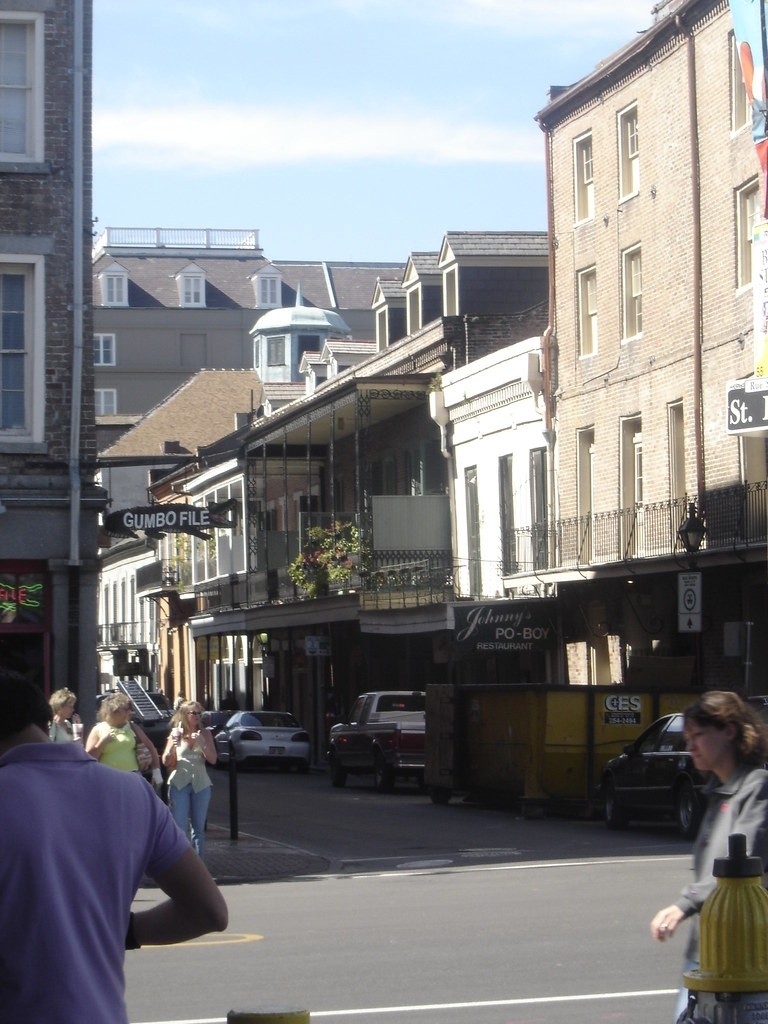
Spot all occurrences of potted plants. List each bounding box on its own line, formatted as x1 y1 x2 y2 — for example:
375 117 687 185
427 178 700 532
286 520 370 599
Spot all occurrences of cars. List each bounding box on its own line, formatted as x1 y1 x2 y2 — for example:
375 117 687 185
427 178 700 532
215 710 310 774
199 710 235 737
603 712 712 838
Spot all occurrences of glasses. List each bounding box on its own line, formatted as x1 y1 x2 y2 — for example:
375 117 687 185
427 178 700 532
184 711 202 716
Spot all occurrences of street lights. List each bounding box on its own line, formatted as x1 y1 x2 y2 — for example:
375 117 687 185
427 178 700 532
678 503 711 690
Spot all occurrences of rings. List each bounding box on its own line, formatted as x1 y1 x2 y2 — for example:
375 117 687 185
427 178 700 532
660 923 669 928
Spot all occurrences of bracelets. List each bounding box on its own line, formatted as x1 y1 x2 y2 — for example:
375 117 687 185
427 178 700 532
126 912 141 950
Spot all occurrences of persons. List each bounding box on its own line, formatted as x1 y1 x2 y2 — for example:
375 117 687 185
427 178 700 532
326 688 346 723
220 690 239 710
47 689 164 794
0 667 229 1024
652 691 768 1023
162 691 216 859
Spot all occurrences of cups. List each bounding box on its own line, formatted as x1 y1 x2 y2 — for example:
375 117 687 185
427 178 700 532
72 724 83 743
172 727 183 747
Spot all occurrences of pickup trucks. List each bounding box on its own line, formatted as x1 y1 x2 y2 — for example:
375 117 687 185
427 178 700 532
96 692 177 748
325 687 425 793
